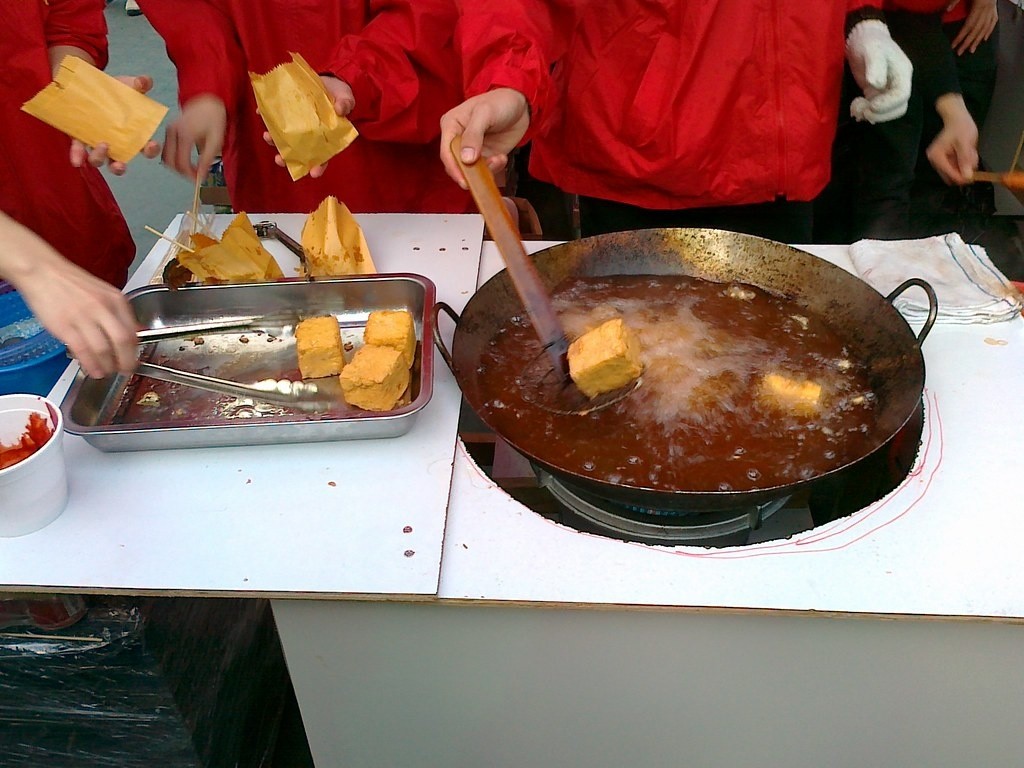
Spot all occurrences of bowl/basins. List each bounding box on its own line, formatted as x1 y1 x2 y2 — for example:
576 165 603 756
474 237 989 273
0 394 69 538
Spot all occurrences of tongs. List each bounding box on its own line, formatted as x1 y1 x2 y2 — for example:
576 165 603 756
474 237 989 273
163 220 315 288
66 308 350 414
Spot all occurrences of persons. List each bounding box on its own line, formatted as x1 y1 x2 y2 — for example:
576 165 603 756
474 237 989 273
0 1 161 397
440 0 913 242
133 0 480 215
884 0 1001 236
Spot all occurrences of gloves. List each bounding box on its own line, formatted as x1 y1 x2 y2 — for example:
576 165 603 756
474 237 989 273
846 19 914 125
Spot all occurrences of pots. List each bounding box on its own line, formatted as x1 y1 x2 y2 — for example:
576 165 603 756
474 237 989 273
430 227 937 513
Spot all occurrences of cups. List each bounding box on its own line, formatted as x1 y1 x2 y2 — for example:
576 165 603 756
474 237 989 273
0 276 73 398
